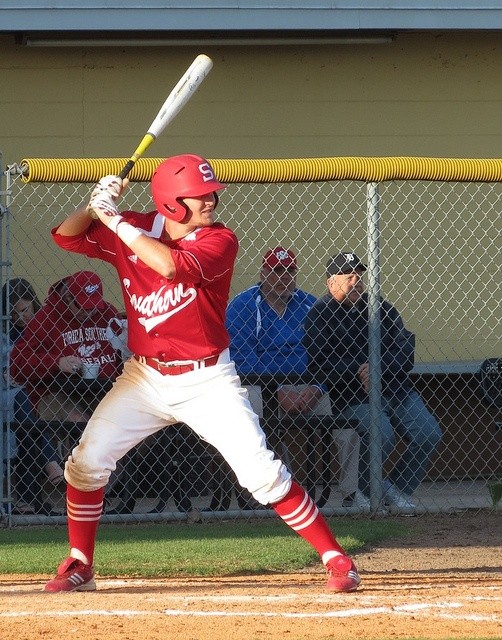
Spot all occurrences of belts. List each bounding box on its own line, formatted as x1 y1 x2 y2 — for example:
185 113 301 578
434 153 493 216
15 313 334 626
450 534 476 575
128 352 229 375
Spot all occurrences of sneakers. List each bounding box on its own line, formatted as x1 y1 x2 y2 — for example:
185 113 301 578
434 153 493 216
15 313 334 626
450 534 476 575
327 556 361 592
382 478 418 517
45 558 97 593
342 493 372 506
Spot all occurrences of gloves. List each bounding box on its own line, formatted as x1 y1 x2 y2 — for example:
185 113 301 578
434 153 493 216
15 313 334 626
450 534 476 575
86 175 129 210
86 193 127 234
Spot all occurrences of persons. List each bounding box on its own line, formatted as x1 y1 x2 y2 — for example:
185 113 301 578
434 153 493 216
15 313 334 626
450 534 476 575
304 251 442 509
9 271 123 487
44 154 363 597
3 279 41 343
225 248 323 415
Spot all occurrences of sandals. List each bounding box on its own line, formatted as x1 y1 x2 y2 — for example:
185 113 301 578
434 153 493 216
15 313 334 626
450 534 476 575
16 502 35 514
50 473 64 484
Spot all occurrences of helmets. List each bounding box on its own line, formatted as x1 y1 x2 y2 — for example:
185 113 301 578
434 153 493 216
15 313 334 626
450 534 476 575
152 154 228 222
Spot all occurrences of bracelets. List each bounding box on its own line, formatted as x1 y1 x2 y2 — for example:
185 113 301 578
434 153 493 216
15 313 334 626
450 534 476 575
107 214 142 247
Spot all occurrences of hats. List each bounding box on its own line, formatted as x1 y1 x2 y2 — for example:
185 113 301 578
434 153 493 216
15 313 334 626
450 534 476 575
326 253 367 278
263 247 297 271
67 270 105 310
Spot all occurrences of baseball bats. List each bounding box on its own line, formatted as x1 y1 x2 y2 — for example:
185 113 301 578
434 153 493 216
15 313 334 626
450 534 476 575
116 54 216 180
